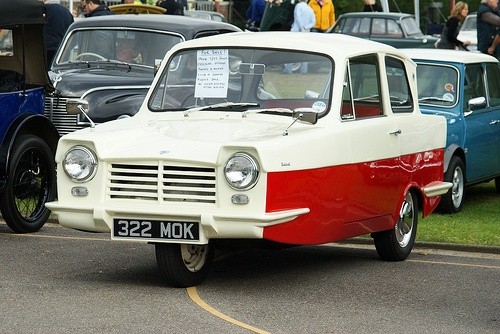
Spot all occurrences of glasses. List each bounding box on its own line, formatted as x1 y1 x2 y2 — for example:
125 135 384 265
83 3 87 9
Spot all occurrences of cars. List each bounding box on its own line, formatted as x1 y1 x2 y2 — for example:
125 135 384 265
183 10 226 23
45 14 276 135
454 11 480 50
283 12 441 76
1 0 74 234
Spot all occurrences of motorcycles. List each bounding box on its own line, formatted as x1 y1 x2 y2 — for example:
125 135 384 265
44 32 454 288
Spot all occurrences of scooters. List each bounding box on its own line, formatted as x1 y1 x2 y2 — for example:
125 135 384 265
399 48 500 213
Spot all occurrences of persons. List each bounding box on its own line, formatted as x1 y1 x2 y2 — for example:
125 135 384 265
81 0 114 60
114 38 142 64
43 0 75 70
476 0 499 58
245 0 336 33
437 2 470 51
